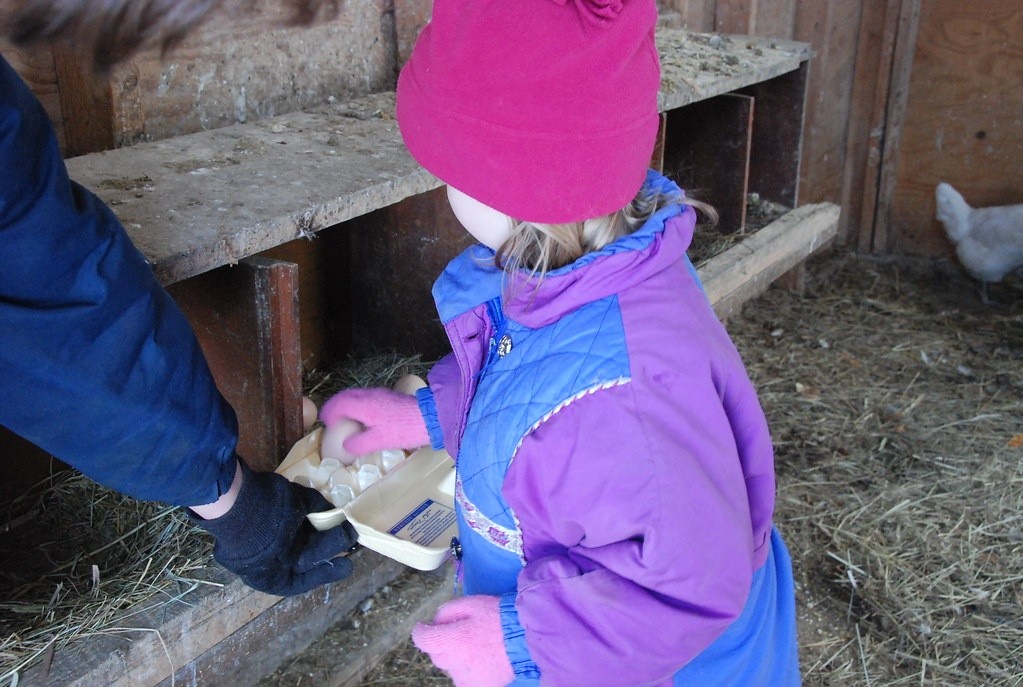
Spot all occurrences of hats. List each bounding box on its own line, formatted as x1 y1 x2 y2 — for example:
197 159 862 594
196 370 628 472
397 0 664 224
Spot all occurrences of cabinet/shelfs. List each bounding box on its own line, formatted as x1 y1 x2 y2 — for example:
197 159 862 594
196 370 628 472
70 22 823 430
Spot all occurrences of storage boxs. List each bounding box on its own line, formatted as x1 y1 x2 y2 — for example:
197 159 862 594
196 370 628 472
277 419 459 572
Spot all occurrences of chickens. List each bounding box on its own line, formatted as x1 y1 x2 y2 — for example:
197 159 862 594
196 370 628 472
934 183 1023 305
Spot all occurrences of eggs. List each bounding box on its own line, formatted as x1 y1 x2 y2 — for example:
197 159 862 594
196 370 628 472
302 374 429 466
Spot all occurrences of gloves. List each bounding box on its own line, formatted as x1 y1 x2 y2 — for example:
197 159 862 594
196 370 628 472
412 595 517 687
185 453 359 596
320 387 431 455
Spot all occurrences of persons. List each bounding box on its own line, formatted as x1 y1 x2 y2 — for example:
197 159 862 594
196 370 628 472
318 0 799 686
0 56 359 598
